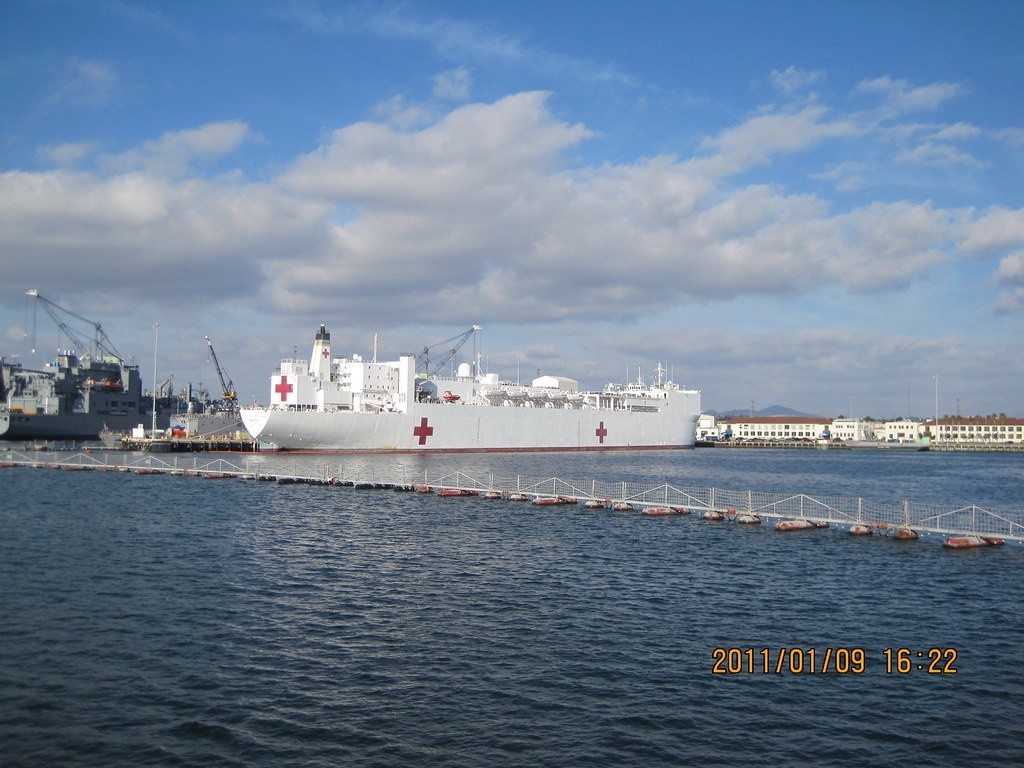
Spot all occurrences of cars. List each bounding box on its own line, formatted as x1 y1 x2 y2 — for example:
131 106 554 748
736 437 812 442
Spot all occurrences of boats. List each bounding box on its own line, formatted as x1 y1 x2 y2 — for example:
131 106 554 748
100 333 262 454
239 325 702 450
0 289 223 440
